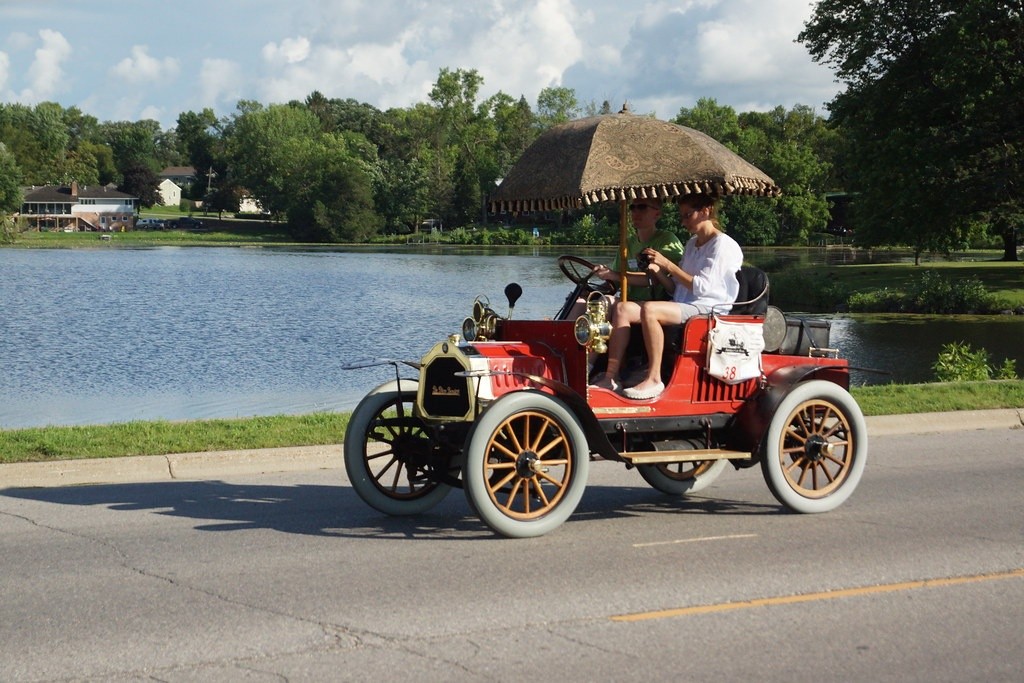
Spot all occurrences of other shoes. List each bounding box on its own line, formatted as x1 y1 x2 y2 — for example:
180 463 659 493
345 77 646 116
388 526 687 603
623 382 665 399
589 380 622 393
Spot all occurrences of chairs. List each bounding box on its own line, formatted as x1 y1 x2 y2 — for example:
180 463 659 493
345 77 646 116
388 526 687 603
652 265 770 389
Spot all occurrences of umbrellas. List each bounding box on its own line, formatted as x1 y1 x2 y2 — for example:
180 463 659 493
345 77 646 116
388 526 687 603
486 102 783 216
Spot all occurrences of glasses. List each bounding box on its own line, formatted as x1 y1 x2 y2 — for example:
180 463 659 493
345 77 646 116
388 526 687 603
682 209 696 219
629 204 658 211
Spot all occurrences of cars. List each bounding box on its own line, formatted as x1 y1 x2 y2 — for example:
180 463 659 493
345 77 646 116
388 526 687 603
154 220 178 230
341 255 869 538
179 217 203 229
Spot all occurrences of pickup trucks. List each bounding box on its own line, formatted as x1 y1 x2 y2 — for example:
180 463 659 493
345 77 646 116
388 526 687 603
136 219 164 230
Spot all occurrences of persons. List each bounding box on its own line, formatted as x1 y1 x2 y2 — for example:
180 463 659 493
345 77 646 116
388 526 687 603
589 191 743 400
583 184 684 376
